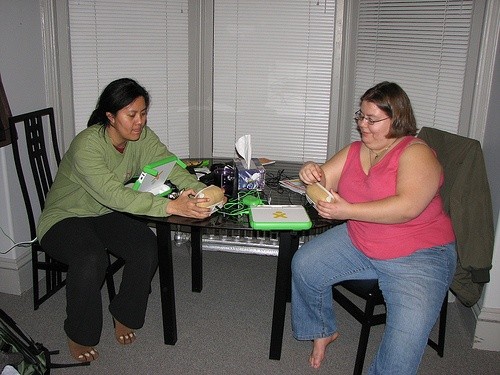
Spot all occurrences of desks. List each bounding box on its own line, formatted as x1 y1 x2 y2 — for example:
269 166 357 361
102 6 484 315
125 161 347 361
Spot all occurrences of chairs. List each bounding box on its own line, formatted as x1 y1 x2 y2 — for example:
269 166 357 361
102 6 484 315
10 108 126 328
332 126 479 375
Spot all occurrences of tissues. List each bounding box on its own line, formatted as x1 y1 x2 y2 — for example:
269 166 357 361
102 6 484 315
232 134 266 191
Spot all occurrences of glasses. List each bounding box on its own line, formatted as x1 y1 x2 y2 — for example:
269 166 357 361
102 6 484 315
354 109 390 125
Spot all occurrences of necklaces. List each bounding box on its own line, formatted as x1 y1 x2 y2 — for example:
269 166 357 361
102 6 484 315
367 137 398 166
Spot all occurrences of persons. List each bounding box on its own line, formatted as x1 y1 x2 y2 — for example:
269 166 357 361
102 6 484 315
36 77 227 361
290 81 458 375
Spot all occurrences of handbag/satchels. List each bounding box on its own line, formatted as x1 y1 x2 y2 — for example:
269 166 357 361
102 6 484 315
0 308 50 374
